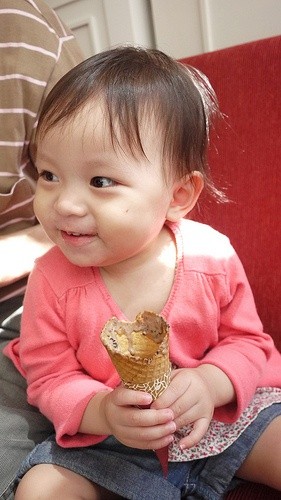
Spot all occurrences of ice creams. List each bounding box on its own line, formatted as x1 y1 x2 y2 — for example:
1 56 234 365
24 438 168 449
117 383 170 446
101 311 172 476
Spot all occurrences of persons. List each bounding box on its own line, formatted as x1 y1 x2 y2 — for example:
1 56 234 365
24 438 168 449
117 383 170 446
0 0 90 500
4 41 281 500
